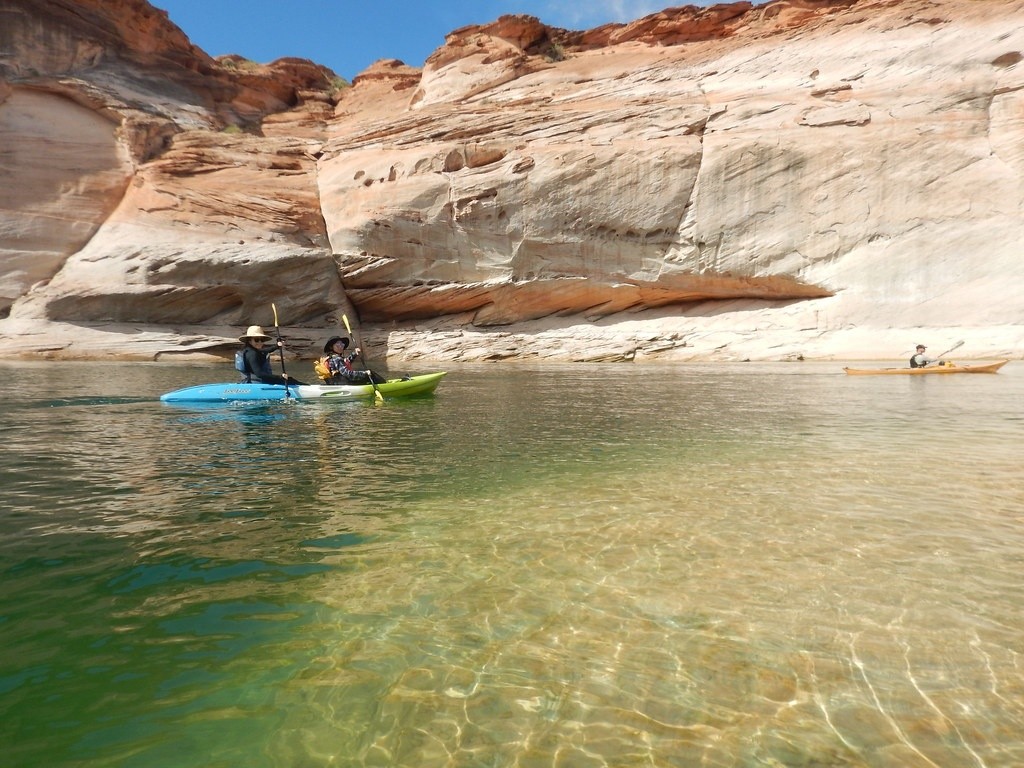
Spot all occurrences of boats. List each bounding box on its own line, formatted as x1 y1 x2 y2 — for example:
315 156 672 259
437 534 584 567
158 368 449 404
840 359 1013 374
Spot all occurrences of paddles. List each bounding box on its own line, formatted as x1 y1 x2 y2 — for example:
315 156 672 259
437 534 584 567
271 302 292 399
342 314 384 402
937 338 966 358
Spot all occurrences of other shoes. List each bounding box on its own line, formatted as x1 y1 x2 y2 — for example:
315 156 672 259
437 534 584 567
402 373 410 381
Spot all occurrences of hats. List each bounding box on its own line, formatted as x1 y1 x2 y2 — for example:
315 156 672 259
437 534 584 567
916 344 927 349
324 336 349 353
239 325 272 342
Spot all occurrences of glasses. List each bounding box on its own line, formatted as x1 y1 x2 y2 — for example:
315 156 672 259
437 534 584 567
334 343 345 347
252 337 264 342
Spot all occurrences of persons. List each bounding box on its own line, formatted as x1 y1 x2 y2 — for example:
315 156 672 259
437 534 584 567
910 345 942 369
324 336 411 386
239 326 309 386
